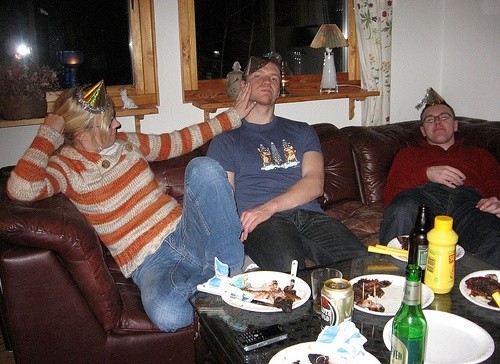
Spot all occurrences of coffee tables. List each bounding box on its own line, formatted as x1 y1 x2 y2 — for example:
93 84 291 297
189 250 500 364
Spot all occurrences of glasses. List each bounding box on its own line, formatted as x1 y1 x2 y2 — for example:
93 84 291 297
422 112 455 126
83 110 117 130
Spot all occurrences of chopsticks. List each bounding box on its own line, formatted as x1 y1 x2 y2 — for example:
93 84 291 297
367 244 408 258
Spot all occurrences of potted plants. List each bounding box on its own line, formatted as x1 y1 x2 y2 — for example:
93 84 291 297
0 63 63 121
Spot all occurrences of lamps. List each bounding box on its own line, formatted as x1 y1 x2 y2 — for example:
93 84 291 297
309 24 349 94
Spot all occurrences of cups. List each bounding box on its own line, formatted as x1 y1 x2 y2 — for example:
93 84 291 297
311 268 343 315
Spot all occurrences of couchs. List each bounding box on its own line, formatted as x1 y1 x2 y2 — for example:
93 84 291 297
0 117 500 364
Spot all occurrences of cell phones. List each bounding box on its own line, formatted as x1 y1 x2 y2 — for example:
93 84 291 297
236 324 288 352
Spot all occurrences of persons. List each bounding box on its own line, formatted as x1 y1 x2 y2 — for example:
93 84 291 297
5 79 259 334
206 55 371 273
378 88 500 270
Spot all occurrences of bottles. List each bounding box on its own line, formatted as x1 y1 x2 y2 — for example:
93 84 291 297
423 215 459 295
388 264 427 364
403 205 433 283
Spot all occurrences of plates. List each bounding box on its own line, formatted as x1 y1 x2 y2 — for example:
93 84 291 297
459 269 500 312
386 234 465 267
221 270 312 313
268 340 381 364
349 274 434 316
382 309 496 364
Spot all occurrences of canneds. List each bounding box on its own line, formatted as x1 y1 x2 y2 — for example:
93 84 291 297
320 278 354 332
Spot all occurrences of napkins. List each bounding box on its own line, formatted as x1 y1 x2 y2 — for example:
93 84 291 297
207 257 244 288
316 316 367 358
